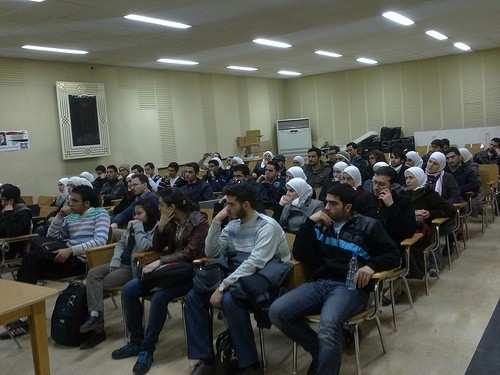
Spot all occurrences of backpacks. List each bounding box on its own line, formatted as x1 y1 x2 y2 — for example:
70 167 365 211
51 281 92 347
216 330 239 375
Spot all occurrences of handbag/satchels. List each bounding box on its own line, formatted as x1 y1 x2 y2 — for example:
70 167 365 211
28 234 69 256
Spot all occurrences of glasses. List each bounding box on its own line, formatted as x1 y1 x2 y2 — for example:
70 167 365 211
368 156 377 161
130 182 142 189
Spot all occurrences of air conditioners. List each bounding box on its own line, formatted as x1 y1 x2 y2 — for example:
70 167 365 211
276 118 312 155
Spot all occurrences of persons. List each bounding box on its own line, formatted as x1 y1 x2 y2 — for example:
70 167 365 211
182 182 291 375
269 183 401 375
0 134 6 146
0 185 111 340
0 183 32 279
111 187 210 375
36 137 500 307
79 198 160 349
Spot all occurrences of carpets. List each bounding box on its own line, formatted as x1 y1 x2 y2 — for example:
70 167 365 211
464 298 500 375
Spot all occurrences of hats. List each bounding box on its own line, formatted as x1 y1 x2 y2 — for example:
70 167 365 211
336 150 351 161
119 164 130 174
1 186 21 201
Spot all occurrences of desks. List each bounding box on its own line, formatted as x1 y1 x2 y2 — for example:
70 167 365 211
244 158 263 173
0 278 60 375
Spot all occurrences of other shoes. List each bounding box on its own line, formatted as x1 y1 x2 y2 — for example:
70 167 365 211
80 329 106 349
479 208 487 215
429 268 440 276
112 342 141 359
454 228 463 240
133 350 153 374
442 243 454 256
239 361 265 375
381 295 392 305
394 290 406 304
190 356 216 375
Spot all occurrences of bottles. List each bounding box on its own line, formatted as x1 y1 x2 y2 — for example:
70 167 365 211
345 252 359 291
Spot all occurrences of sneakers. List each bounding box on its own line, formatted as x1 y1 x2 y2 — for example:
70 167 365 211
6 316 29 327
0 323 30 340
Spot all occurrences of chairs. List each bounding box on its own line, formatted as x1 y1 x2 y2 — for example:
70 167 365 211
0 144 500 375
236 130 263 159
